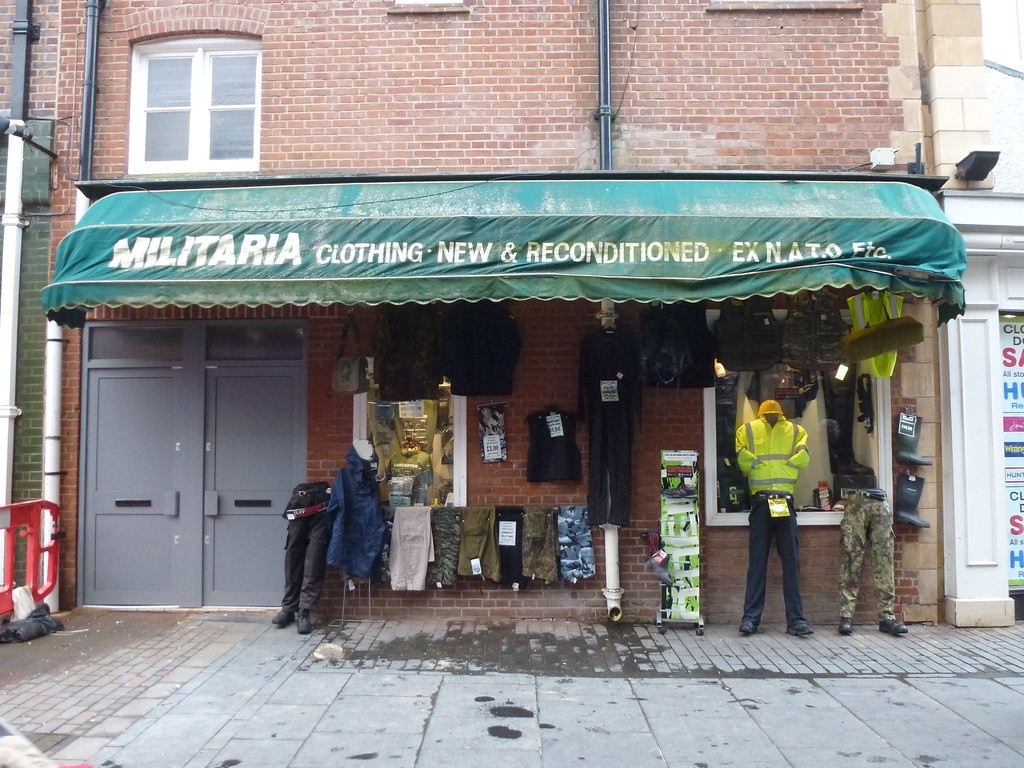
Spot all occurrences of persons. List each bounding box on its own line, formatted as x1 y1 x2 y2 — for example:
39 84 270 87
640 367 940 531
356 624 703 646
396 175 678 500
272 481 334 634
819 419 841 473
837 487 909 633
735 400 814 637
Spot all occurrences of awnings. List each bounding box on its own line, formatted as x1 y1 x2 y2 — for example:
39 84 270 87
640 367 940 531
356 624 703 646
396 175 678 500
42 181 969 332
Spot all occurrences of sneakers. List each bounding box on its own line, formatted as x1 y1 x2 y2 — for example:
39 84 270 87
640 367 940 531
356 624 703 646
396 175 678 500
271 607 295 625
298 608 311 634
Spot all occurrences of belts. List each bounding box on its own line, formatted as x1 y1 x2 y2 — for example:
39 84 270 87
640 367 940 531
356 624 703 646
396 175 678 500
751 492 793 502
847 490 887 501
293 489 315 496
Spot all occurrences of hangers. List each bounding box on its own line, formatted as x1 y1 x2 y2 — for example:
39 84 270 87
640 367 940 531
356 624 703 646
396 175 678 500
476 399 511 411
584 315 638 343
846 286 903 303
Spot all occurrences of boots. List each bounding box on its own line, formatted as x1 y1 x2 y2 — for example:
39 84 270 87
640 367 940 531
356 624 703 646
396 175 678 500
821 363 874 475
895 413 933 466
895 473 931 528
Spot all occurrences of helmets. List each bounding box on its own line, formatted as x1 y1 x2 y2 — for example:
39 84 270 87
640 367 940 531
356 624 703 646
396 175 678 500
758 400 783 415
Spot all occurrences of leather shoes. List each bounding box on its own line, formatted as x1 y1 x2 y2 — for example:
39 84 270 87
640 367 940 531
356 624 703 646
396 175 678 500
838 617 852 634
785 623 814 635
879 620 908 634
739 619 757 634
